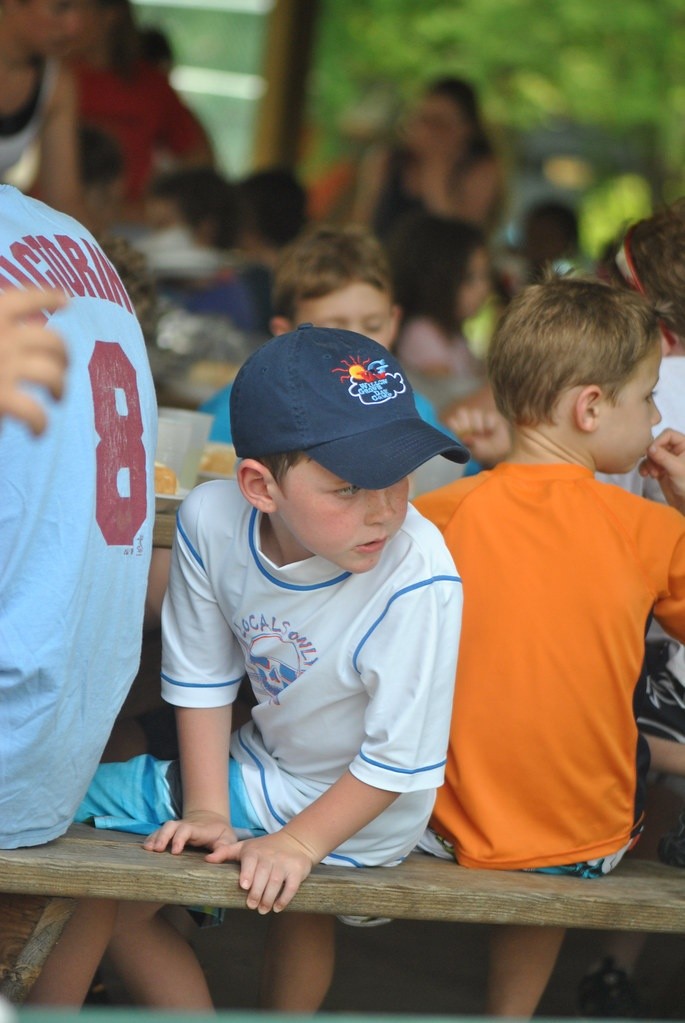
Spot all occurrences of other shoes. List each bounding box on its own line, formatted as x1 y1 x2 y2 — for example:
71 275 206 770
576 955 647 1018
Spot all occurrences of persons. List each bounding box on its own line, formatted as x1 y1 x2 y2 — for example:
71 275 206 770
0 182 159 1012
411 277 685 1023
445 212 685 743
110 222 482 761
0 0 581 360
75 321 471 1016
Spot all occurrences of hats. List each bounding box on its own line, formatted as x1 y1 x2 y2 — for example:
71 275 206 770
229 324 470 489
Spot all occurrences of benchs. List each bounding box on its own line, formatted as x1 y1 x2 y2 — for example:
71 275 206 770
0 821 685 1004
